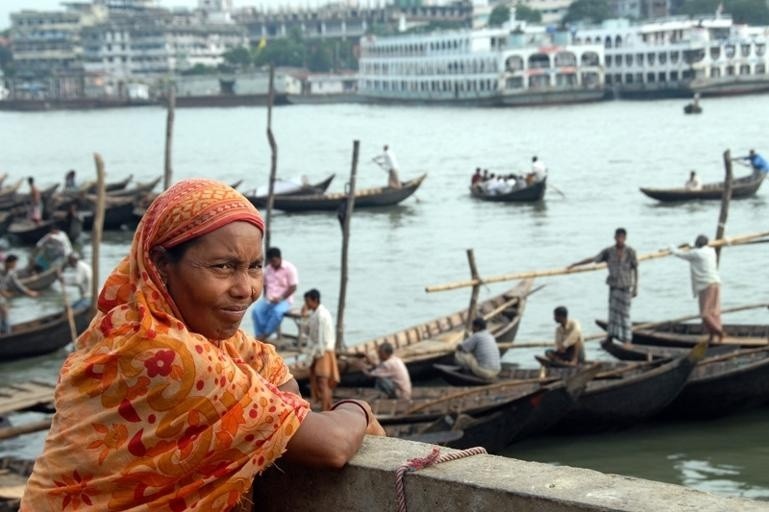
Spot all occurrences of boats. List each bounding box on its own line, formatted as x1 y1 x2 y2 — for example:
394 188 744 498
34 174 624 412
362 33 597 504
684 104 703 114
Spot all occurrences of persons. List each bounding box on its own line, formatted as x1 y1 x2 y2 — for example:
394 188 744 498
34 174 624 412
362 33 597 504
372 144 400 187
685 169 700 187
743 148 768 178
297 289 343 412
352 342 413 398
0 169 94 318
667 233 727 347
469 153 542 199
543 306 588 368
564 226 641 348
252 243 296 344
21 178 389 511
454 317 502 379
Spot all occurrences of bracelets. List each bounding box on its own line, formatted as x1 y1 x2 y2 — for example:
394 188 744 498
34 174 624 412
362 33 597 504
331 398 371 428
